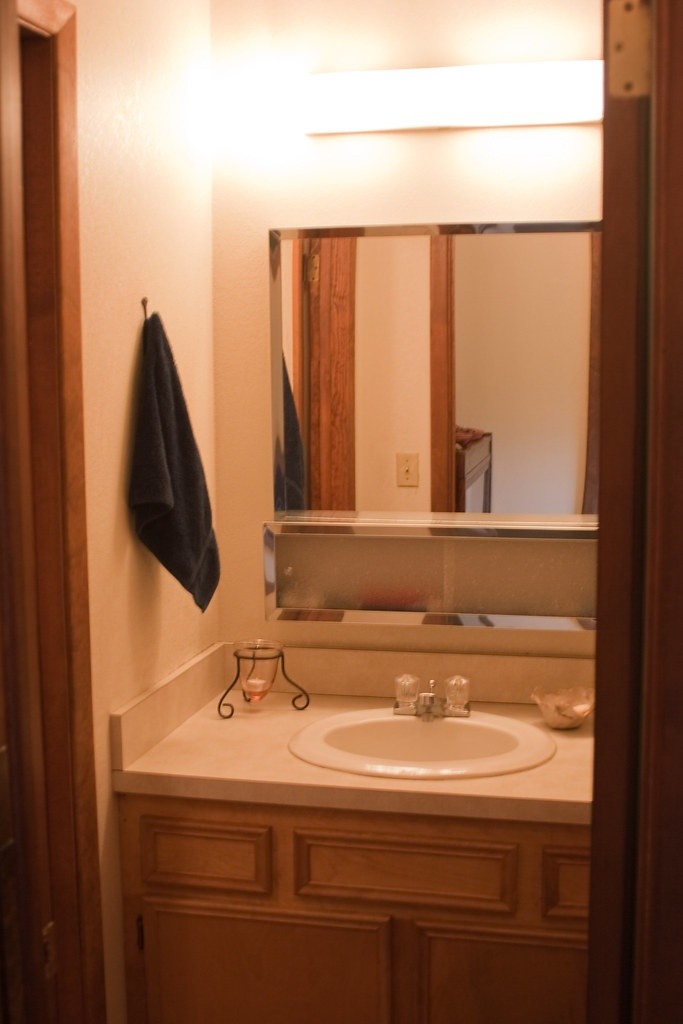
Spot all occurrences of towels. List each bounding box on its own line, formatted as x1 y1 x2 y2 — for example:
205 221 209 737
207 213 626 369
125 311 221 614
282 349 305 512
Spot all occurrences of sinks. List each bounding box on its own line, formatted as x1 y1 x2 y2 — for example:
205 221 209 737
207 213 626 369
287 706 558 779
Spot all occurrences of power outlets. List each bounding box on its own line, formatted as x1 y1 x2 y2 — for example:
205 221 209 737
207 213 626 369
396 451 419 487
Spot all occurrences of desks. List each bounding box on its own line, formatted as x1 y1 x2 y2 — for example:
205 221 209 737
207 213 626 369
455 433 493 513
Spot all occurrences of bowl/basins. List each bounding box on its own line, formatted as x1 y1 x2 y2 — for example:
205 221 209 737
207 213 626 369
530 685 595 729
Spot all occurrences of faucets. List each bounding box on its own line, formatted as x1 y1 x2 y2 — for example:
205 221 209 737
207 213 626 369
394 672 470 723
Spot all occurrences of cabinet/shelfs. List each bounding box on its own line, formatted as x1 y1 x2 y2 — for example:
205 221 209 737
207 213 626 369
118 792 590 1024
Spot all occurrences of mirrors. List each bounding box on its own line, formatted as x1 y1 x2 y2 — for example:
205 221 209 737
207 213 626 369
270 219 599 524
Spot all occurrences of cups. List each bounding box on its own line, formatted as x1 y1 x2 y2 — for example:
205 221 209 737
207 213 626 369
235 639 282 703
